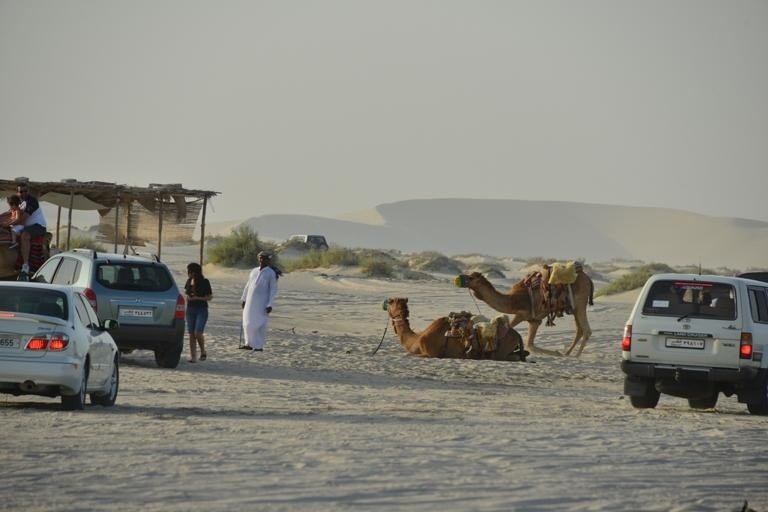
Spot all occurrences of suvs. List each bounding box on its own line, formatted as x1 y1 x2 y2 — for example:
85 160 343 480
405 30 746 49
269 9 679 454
17 245 187 368
283 233 330 253
619 265 767 419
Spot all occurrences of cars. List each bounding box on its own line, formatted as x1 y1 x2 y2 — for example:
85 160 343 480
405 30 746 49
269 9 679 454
0 279 122 412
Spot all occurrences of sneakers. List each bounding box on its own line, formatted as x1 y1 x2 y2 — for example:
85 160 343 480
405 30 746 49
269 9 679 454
17 271 30 281
9 242 18 249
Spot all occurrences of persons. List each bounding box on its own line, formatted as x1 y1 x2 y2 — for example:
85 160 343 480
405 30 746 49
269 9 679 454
15 180 47 281
6 193 24 251
181 262 214 364
237 250 279 352
